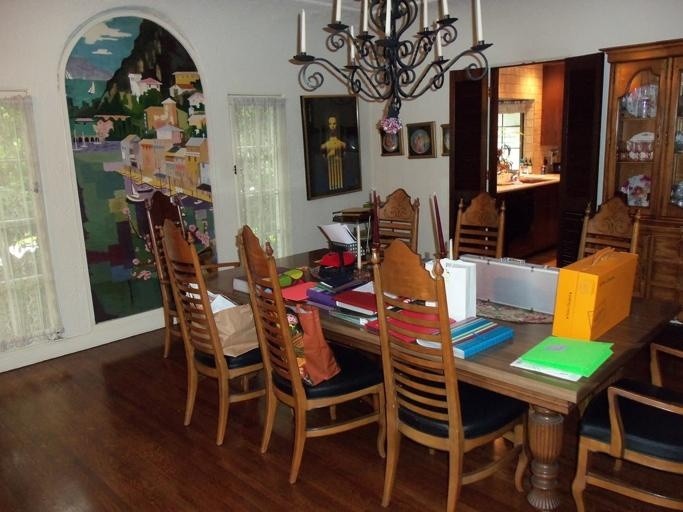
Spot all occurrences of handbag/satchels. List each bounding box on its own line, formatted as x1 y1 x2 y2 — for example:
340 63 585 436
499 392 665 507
257 300 342 387
425 258 478 321
184 283 260 358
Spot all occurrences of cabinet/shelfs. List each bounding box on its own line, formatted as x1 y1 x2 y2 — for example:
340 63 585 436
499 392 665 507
595 36 683 232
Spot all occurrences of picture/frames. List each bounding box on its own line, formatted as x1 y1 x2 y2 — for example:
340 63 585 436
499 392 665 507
377 122 405 159
299 93 365 202
405 121 437 160
440 120 450 160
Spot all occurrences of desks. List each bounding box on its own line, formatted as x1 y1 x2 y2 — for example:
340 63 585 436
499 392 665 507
180 247 682 511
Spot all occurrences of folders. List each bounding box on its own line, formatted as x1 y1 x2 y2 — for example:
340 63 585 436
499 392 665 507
365 309 456 347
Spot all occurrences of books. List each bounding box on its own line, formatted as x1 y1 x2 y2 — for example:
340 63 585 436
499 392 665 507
232 264 304 298
280 266 408 327
508 334 614 383
316 220 367 259
364 303 456 346
415 314 514 361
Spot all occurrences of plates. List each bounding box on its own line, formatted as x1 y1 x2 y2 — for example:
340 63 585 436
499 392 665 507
621 175 652 208
626 85 659 118
625 131 656 162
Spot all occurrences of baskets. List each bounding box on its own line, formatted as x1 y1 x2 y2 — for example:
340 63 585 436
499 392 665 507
328 240 366 259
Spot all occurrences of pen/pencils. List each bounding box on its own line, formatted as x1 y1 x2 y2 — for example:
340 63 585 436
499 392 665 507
320 281 333 288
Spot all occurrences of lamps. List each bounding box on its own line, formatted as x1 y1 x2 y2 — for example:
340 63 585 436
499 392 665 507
292 1 497 138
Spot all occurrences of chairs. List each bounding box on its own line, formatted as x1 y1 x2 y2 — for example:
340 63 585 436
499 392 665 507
235 225 385 484
143 191 185 359
371 188 421 254
368 236 530 508
567 341 682 511
451 191 509 260
154 219 266 448
573 195 642 261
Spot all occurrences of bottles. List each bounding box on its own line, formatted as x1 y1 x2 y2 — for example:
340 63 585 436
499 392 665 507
635 90 650 119
527 158 533 173
523 160 527 167
